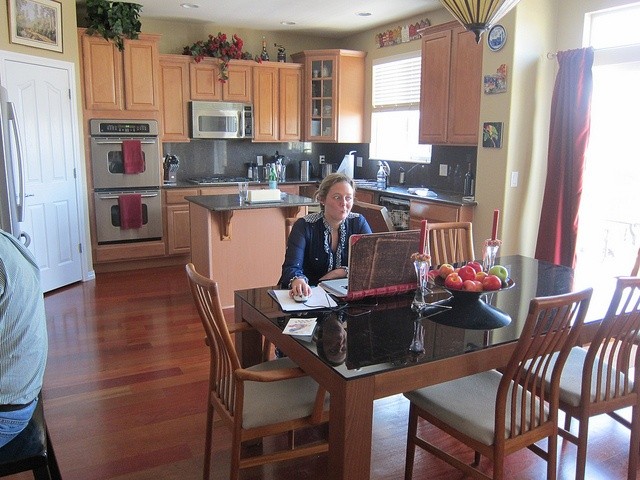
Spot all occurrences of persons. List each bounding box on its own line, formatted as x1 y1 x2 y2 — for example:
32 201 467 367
275 173 373 359
0 229 49 448
291 303 348 367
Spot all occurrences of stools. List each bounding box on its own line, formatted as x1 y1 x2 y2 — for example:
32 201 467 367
1 392 63 479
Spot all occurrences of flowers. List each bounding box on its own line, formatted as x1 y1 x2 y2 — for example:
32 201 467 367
180 29 262 84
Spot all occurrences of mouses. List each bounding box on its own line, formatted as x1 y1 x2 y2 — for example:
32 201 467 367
292 291 309 302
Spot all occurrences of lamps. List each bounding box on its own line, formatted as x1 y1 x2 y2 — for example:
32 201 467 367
436 1 522 44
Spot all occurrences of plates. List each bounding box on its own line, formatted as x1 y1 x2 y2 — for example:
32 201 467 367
420 300 512 331
433 273 516 301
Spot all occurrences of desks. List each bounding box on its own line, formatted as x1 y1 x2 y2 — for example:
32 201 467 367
234 254 639 480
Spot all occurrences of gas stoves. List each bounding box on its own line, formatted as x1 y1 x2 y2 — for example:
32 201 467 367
187 176 251 184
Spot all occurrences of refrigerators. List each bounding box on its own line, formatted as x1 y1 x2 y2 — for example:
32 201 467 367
0 86 30 267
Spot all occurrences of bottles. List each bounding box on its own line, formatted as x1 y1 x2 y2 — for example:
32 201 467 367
321 163 334 178
376 162 387 189
461 163 476 198
281 164 287 185
253 163 260 182
300 159 310 182
268 166 277 191
248 166 253 179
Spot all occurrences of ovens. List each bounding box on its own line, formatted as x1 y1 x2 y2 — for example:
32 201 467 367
88 120 162 246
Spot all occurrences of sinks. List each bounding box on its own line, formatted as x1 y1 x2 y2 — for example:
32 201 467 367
353 179 378 184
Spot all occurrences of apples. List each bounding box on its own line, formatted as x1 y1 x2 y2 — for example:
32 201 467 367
474 281 483 291
483 275 502 290
488 266 507 280
476 272 488 282
455 268 461 272
458 266 475 282
467 261 483 273
444 273 463 289
439 264 454 278
462 280 476 292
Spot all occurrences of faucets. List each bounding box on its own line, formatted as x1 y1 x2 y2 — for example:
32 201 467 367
382 160 391 188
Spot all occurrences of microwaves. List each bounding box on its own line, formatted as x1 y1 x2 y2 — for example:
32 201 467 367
191 102 254 138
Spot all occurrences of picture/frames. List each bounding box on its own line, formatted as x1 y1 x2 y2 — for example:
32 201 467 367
7 0 64 54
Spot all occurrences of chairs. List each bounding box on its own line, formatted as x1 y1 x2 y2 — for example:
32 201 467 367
184 262 333 479
421 221 475 270
499 275 639 477
612 248 640 375
403 290 592 478
284 217 298 246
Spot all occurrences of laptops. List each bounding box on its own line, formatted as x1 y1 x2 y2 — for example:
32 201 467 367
320 230 423 300
345 301 418 370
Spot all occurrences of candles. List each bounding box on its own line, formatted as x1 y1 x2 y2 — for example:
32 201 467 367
419 219 427 255
491 209 498 241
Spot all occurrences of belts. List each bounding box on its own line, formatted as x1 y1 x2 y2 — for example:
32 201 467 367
0 398 35 413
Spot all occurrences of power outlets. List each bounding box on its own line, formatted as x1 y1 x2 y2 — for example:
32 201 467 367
356 157 363 167
319 155 327 164
439 164 448 176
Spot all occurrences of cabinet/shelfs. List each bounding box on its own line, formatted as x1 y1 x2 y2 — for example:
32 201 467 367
252 62 301 142
356 191 379 207
189 57 253 104
159 53 190 144
199 187 300 199
406 197 474 241
78 27 160 120
303 49 367 142
417 18 484 146
162 189 200 257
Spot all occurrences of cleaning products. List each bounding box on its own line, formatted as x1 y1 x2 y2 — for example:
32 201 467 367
375 163 388 191
269 168 277 190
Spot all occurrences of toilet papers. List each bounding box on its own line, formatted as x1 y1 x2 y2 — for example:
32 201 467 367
337 155 355 180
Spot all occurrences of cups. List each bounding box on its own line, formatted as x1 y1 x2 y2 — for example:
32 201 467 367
237 184 250 202
